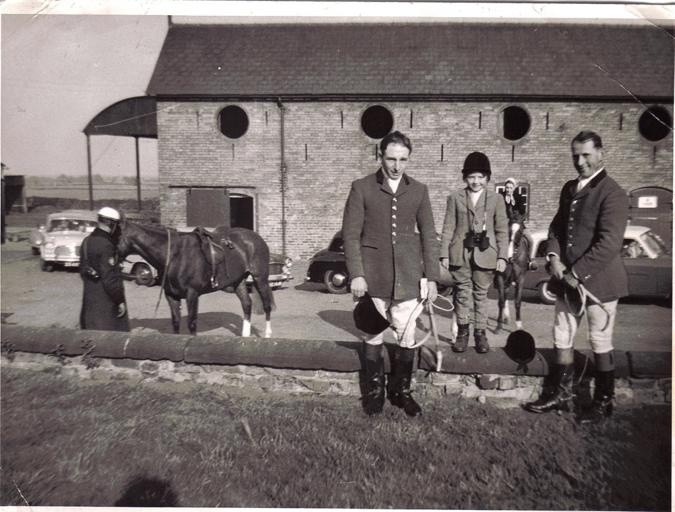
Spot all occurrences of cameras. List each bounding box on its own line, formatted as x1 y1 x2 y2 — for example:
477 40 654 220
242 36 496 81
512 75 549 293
83 266 101 283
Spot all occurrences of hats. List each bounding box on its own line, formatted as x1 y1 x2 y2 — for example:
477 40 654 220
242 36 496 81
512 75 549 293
506 178 516 185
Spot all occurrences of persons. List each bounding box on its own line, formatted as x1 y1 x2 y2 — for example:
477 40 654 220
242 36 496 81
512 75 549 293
621 239 642 258
438 152 512 353
526 130 631 424
342 130 441 417
78 207 132 331
502 176 526 259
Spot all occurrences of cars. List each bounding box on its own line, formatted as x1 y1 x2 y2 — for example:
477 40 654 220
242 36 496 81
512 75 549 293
30 209 102 274
297 223 456 297
118 224 297 293
514 224 671 304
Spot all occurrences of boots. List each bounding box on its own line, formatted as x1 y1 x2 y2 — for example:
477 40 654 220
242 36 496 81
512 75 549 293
579 370 615 423
474 329 489 352
527 362 575 412
391 359 422 416
357 352 384 414
453 323 469 352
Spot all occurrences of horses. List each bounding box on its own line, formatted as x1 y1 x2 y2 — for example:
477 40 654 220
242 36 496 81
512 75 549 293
110 209 277 338
495 205 530 333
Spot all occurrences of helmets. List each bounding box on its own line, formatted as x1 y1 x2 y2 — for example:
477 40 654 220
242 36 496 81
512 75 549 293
353 292 388 334
99 207 120 221
506 329 534 373
463 152 490 176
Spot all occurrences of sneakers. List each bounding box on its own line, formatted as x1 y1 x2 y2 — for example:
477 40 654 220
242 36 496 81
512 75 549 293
528 258 537 270
